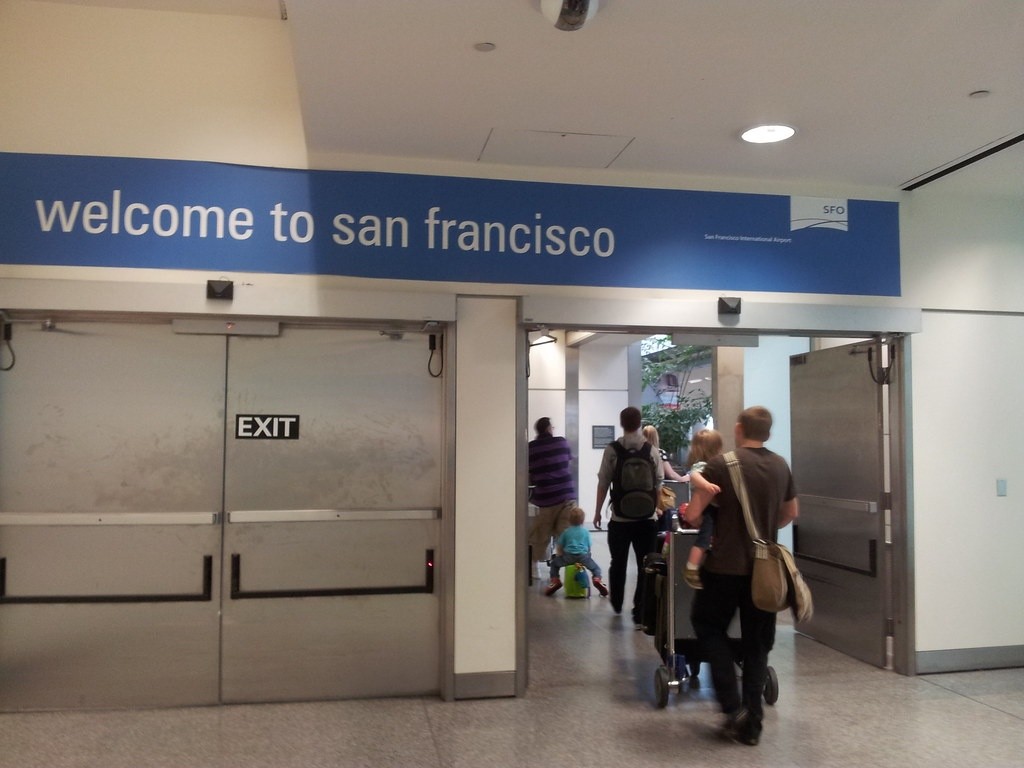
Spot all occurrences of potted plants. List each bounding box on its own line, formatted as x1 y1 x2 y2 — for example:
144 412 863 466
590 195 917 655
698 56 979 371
641 335 713 483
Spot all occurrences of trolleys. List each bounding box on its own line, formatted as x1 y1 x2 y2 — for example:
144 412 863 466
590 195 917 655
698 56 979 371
643 527 779 709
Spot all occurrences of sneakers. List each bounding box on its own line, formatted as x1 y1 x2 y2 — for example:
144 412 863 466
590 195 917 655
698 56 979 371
681 566 703 589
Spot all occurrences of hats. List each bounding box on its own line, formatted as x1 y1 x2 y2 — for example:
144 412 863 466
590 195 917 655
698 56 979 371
576 566 589 588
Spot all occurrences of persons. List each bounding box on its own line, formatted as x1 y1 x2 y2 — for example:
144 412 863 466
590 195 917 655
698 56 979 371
680 430 723 589
528 417 578 579
592 407 691 630
685 405 799 746
544 508 608 597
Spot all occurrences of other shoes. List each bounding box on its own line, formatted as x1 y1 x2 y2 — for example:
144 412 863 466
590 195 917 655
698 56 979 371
721 706 750 731
733 727 760 746
636 624 644 631
545 577 563 597
610 598 622 614
593 577 609 595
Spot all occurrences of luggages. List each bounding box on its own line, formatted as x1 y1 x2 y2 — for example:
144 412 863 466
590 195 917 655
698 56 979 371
563 563 590 599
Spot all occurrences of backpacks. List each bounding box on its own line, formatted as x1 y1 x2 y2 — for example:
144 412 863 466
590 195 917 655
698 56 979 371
608 440 659 520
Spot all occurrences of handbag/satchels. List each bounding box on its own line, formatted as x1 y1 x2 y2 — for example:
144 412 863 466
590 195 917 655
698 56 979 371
656 487 677 511
752 538 814 624
